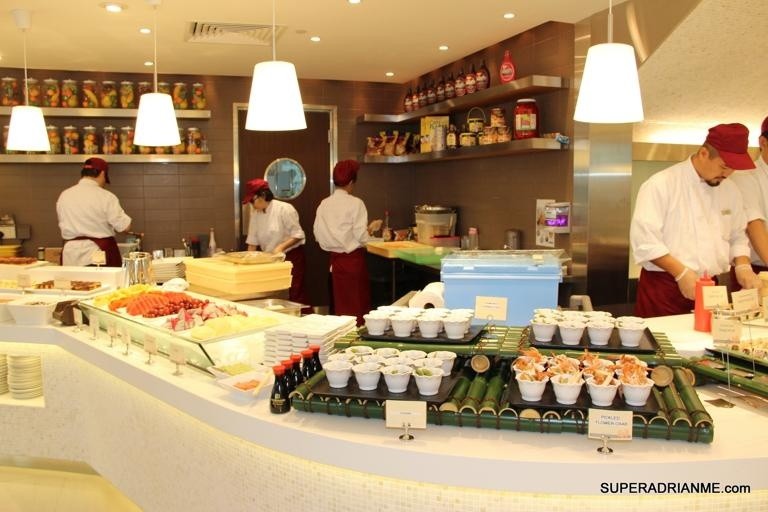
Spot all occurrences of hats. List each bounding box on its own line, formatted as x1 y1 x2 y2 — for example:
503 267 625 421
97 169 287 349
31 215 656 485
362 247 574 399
706 123 756 170
82 158 109 184
333 160 360 186
241 178 268 204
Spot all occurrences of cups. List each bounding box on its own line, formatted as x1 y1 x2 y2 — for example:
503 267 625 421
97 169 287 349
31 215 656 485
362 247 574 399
323 306 476 396
513 307 654 406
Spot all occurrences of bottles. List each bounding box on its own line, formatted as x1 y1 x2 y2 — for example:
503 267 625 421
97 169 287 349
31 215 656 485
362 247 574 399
191 228 216 259
402 50 516 112
37 247 45 261
0 77 207 154
461 227 479 251
694 270 716 333
269 343 322 414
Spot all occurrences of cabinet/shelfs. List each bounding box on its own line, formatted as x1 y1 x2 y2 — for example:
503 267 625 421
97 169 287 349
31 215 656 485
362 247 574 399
0 104 211 165
356 73 570 165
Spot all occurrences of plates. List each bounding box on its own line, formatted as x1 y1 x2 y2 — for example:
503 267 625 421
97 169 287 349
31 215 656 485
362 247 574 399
0 281 358 400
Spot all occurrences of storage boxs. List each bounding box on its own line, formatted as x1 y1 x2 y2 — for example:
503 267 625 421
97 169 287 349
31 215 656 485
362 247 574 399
443 256 563 337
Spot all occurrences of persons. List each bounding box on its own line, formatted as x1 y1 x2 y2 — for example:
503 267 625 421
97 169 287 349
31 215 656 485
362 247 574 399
727 115 768 293
242 179 312 314
55 158 132 267
313 159 383 327
630 123 762 319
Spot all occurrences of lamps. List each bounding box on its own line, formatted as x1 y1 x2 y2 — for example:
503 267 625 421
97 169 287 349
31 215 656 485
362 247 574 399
133 3 181 148
244 6 309 131
572 0 644 125
4 8 52 153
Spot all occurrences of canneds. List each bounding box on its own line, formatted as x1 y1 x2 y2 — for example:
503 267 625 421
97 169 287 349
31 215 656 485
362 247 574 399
478 133 484 146
186 128 202 154
460 133 477 146
484 126 498 145
0 78 19 106
62 79 80 108
468 118 485 133
102 126 118 155
137 81 153 107
41 79 61 107
155 147 170 154
172 82 189 109
80 79 99 107
82 127 99 154
120 126 135 154
490 108 505 127
513 98 541 140
3 125 19 154
498 127 513 143
22 78 41 106
120 81 136 108
63 126 80 154
157 82 171 94
191 83 207 110
45 125 61 154
100 80 118 108
138 146 154 154
171 128 186 154
